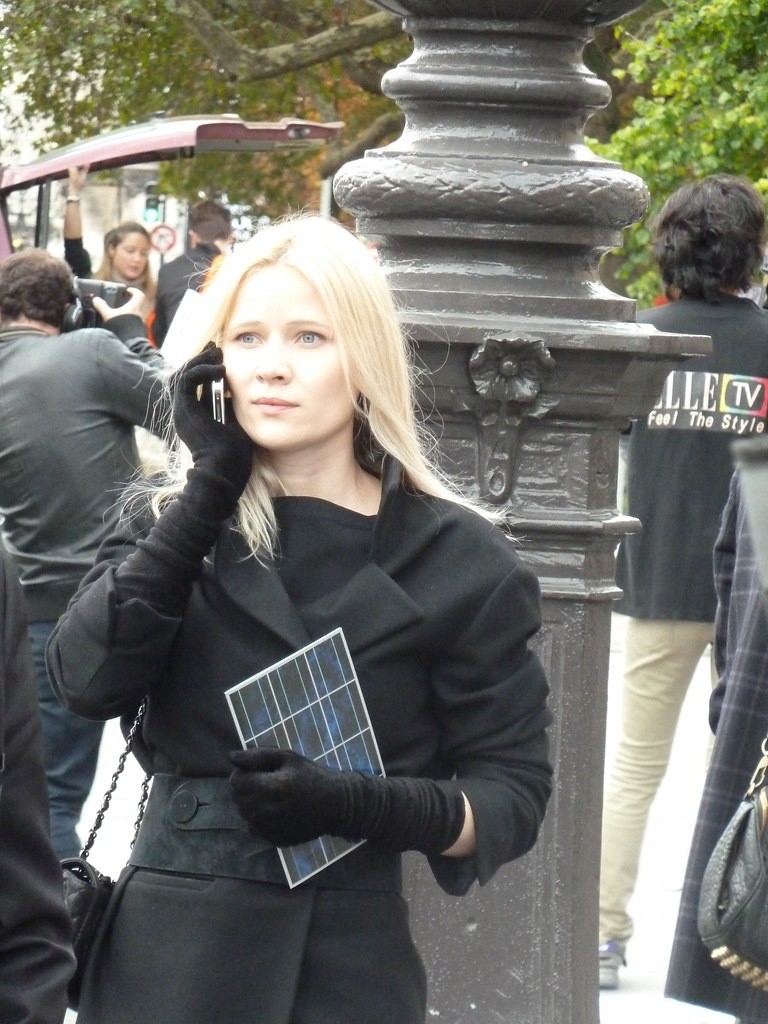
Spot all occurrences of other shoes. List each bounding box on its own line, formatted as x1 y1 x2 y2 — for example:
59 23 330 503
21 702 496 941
599 940 628 987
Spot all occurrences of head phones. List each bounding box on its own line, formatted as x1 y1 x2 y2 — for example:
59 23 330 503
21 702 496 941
61 274 83 333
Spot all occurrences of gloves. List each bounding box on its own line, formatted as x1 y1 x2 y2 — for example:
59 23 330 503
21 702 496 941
231 743 467 855
115 339 251 617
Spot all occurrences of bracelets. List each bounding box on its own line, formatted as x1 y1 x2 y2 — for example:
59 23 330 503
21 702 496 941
66 194 81 203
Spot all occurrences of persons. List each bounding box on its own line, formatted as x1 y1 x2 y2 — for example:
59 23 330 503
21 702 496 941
44 214 555 1024
62 163 238 352
597 173 768 1024
0 238 178 861
0 535 79 1024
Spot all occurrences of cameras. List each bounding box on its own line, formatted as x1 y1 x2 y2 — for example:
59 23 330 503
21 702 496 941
74 278 129 330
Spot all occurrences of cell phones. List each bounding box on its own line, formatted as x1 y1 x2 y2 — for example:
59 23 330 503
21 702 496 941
211 377 225 425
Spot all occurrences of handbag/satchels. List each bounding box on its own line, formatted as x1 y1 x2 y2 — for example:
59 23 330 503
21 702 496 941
697 735 768 992
58 853 116 1010
